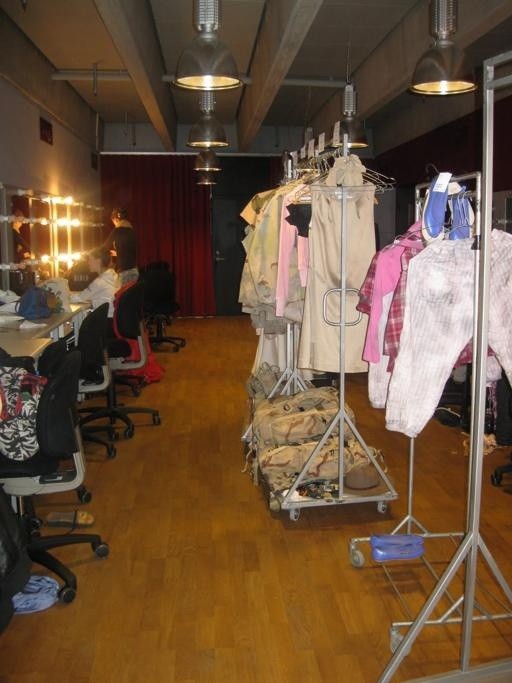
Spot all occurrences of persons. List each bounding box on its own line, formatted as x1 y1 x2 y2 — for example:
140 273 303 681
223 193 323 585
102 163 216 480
67 248 121 339
90 207 139 286
0 349 49 476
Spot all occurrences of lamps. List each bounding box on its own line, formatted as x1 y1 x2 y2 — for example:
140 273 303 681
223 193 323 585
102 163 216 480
171 0 243 184
409 0 479 96
332 28 369 149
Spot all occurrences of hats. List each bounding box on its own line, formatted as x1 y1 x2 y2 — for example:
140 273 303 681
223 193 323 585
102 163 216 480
336 467 393 496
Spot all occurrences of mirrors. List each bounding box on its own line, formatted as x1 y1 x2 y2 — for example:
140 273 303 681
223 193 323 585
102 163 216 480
6 188 107 292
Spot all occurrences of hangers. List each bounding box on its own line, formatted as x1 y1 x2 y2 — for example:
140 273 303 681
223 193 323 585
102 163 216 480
379 190 498 260
279 145 395 200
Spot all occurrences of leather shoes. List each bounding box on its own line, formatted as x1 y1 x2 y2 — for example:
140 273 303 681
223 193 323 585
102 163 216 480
371 535 426 548
449 187 472 241
421 175 448 243
372 544 424 562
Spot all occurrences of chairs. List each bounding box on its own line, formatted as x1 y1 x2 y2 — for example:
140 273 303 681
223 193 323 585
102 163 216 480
491 379 512 487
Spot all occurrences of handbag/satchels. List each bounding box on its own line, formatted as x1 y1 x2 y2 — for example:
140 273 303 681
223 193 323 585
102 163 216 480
253 387 355 446
0 368 48 461
39 278 71 309
258 438 388 492
15 286 53 319
68 260 91 291
247 362 292 397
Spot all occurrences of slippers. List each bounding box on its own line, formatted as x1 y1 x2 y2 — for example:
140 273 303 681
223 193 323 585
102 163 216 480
46 510 94 527
